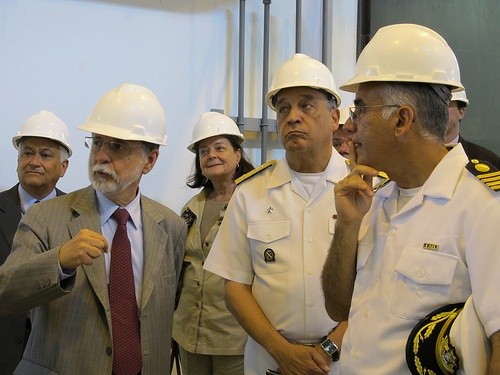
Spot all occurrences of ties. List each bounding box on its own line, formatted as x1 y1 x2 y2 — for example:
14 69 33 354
109 209 143 375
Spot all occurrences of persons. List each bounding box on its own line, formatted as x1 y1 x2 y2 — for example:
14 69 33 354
0 82 189 375
202 23 500 375
1 110 74 374
171 111 256 375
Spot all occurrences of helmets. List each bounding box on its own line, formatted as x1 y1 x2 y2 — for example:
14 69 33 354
335 104 355 124
12 111 72 158
77 82 167 145
451 82 470 108
338 23 465 93
265 53 341 112
187 111 245 153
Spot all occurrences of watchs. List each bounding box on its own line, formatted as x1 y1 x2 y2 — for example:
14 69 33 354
320 336 339 364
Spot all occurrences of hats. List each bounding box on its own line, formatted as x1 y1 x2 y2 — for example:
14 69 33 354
405 294 491 375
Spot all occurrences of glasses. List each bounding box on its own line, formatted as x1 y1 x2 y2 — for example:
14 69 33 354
333 140 352 147
348 105 415 124
83 136 144 156
